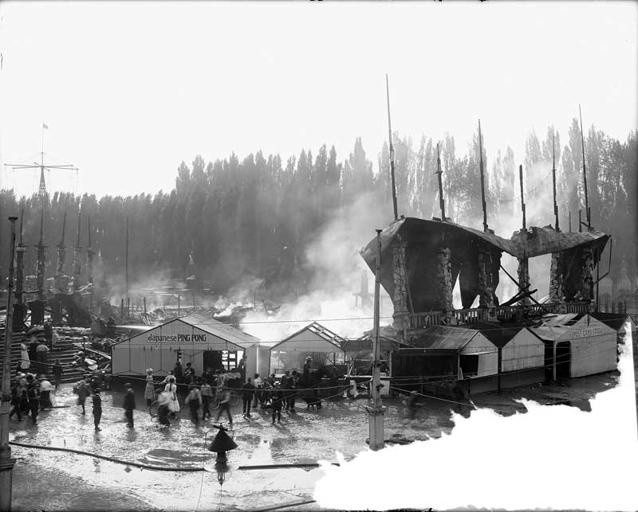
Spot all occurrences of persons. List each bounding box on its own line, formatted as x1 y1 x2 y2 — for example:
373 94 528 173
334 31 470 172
12 338 64 425
123 383 136 428
74 339 112 431
145 362 233 428
237 355 313 424
